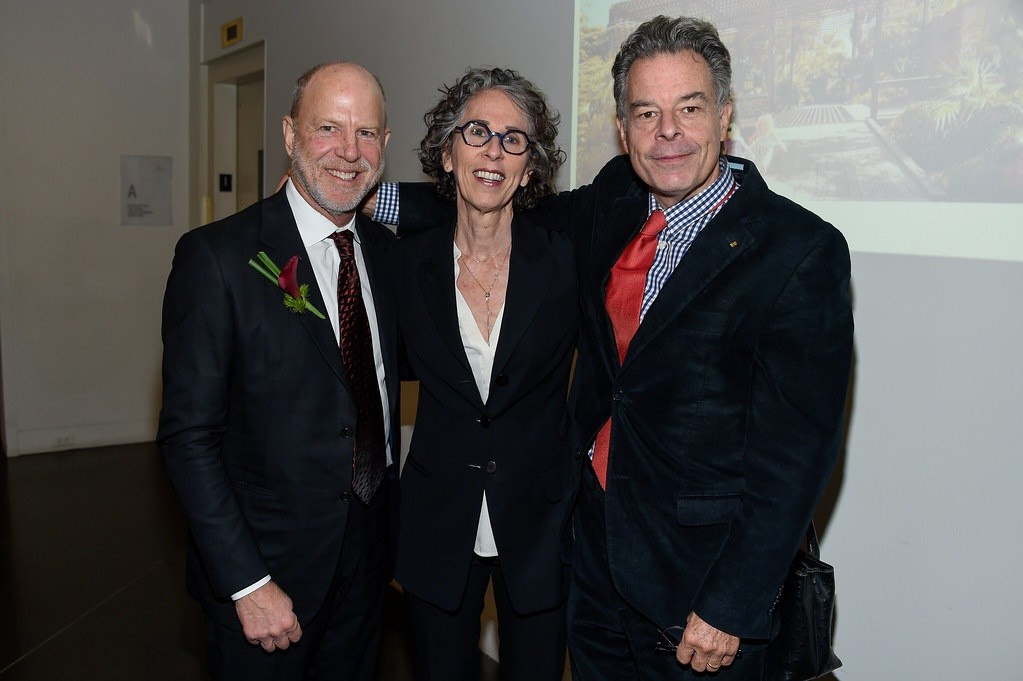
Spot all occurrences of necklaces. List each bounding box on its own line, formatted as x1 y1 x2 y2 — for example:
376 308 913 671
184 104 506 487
457 244 518 345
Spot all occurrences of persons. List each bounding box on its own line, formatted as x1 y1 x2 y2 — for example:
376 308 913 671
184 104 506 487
398 66 582 680
156 61 412 681
361 14 858 678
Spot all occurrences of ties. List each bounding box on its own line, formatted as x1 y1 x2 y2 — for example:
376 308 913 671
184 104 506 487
328 230 388 504
591 209 667 491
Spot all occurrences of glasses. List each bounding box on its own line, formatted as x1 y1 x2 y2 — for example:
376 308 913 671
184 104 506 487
449 121 537 156
